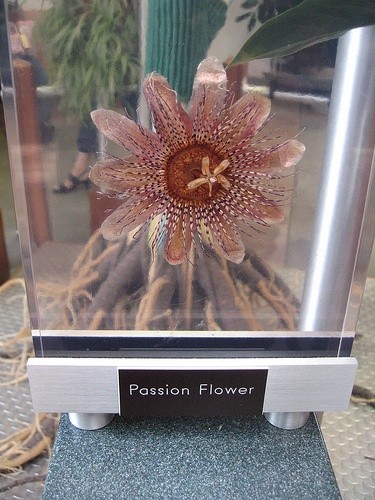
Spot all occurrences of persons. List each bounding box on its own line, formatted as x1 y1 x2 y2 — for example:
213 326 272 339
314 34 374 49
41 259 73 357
6 0 55 143
50 92 139 197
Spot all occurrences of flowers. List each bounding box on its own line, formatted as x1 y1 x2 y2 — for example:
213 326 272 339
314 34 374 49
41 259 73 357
87 55 307 264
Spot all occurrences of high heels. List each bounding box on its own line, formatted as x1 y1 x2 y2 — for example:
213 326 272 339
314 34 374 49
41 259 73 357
53 172 91 194
